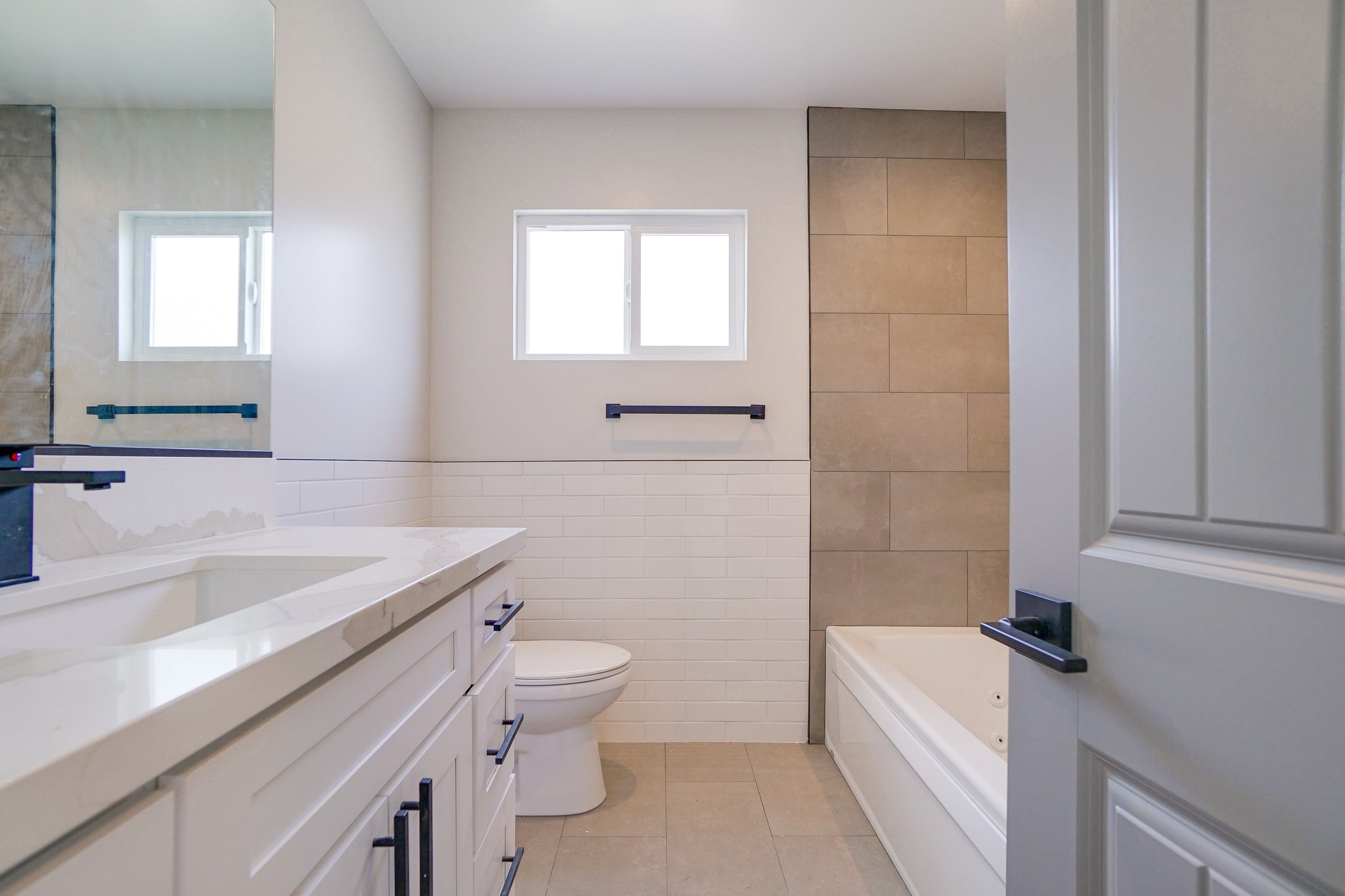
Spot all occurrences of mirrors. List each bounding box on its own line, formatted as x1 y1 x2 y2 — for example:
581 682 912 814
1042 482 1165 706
0 0 277 458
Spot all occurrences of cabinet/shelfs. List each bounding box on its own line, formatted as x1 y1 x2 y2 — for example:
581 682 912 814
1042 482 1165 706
0 570 526 896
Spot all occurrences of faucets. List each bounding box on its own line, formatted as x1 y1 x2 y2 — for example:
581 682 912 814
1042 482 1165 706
0 440 128 587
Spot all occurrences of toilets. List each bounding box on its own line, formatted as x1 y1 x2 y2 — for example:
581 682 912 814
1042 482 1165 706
509 638 634 816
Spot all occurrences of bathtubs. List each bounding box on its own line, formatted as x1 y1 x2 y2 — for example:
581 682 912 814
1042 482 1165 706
821 624 1009 896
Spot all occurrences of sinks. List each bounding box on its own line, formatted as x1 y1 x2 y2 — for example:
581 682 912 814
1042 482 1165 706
1 549 389 652
